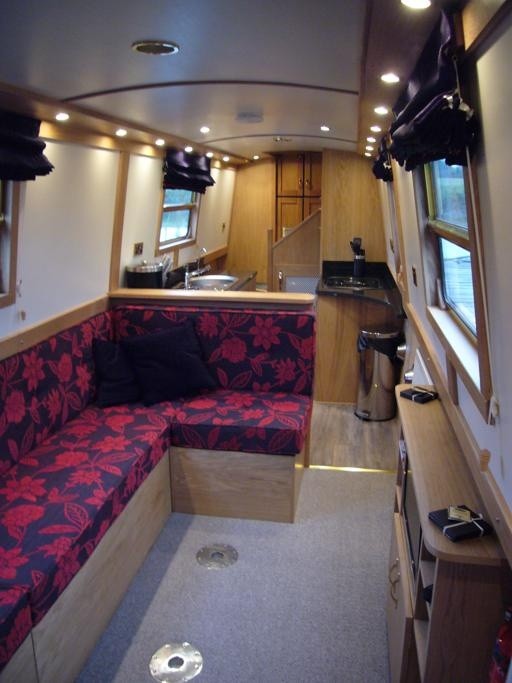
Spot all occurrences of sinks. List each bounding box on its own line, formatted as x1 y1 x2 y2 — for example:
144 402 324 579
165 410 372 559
190 275 239 290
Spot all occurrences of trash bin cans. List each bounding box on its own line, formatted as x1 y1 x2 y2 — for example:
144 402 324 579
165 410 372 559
354 324 403 422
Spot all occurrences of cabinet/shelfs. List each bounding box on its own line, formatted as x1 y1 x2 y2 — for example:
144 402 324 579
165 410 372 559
385 383 508 683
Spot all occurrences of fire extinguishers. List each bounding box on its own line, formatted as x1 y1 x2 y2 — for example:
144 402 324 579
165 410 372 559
487 607 512 683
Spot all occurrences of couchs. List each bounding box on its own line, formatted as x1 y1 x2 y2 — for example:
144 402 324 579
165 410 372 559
0 287 317 683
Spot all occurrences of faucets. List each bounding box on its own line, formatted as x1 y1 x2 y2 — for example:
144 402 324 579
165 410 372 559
197 248 208 272
